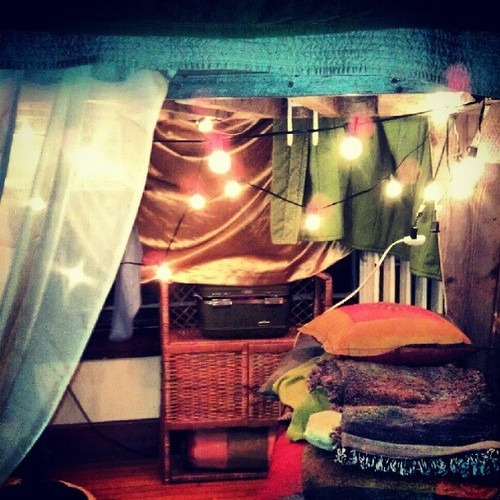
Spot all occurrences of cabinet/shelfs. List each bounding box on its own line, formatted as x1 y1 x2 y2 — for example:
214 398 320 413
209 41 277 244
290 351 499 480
158 266 333 483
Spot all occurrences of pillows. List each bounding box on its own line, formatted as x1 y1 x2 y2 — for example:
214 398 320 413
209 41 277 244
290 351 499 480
296 300 473 361
